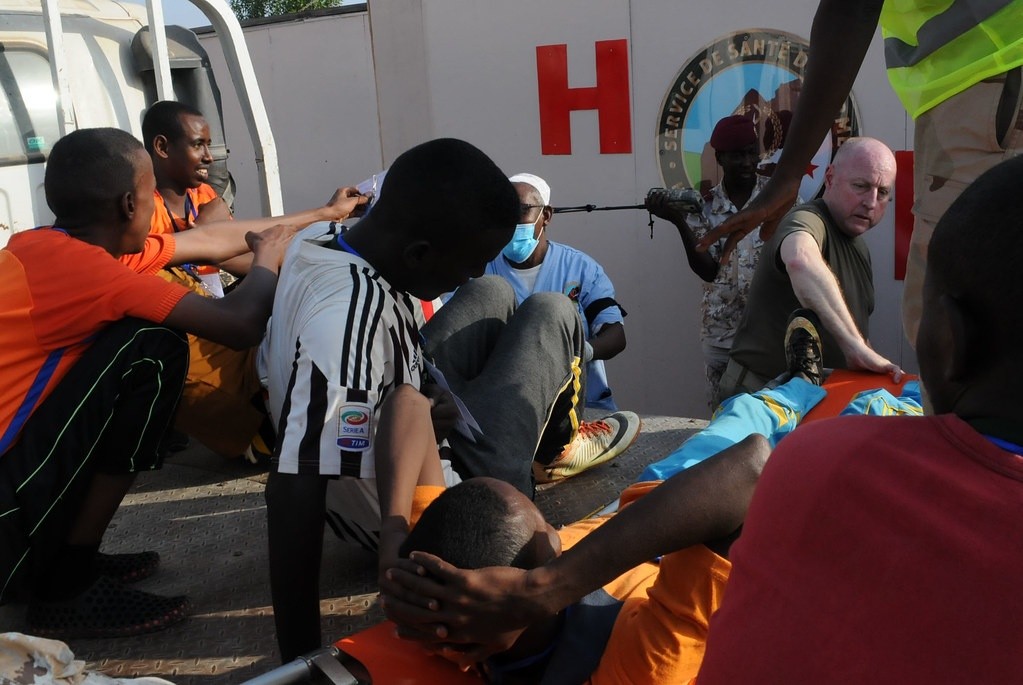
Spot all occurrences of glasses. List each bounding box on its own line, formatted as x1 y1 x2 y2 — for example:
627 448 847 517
520 204 544 215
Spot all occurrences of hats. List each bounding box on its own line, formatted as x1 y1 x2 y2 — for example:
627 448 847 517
509 173 551 207
710 115 759 152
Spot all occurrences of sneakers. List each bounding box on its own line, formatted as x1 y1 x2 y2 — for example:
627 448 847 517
24 573 195 639
532 411 642 492
783 308 826 387
92 550 161 582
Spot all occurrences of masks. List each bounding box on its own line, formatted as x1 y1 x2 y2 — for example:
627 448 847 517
502 207 544 264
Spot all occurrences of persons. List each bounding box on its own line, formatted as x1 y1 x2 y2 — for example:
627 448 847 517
374 1 1023 685
0 100 643 685
717 136 906 407
